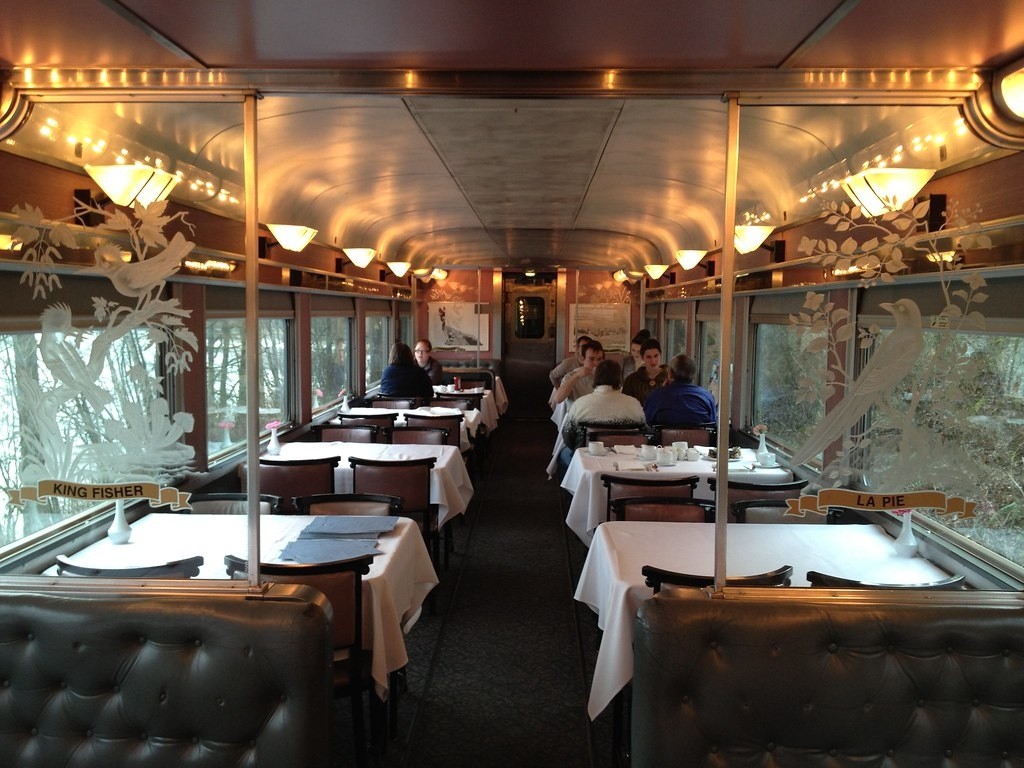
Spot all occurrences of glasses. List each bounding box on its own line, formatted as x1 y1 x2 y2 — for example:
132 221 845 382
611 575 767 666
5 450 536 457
414 349 430 353
667 368 671 372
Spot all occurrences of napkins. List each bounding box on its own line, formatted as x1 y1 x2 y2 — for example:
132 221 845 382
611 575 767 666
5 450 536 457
614 444 636 456
618 458 646 472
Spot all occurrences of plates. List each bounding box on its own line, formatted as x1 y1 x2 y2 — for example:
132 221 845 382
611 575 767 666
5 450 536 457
659 461 676 467
755 461 781 468
694 445 743 462
583 451 610 456
635 455 656 462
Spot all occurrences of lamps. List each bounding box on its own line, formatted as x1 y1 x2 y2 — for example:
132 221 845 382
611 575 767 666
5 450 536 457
85 61 1024 283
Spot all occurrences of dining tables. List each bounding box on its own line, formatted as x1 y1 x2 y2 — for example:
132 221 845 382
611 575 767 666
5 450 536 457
431 375 508 436
44 504 439 759
261 439 474 574
573 513 968 724
329 406 482 452
559 445 794 547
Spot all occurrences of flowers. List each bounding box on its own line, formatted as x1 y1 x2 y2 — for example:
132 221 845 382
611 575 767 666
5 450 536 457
218 421 235 429
266 421 281 430
893 507 912 515
753 425 769 435
315 389 324 397
338 388 347 397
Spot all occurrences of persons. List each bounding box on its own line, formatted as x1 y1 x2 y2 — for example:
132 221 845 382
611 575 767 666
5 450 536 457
379 343 434 398
623 340 668 407
548 335 592 390
414 338 442 386
556 360 648 484
622 329 650 381
556 341 607 412
643 354 718 428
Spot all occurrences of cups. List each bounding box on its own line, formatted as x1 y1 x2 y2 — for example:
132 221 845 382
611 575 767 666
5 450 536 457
475 388 484 393
641 445 662 458
433 385 456 392
657 448 678 464
671 441 698 461
757 453 776 465
589 441 607 454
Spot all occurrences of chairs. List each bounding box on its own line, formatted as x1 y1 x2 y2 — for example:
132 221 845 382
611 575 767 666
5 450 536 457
577 421 967 768
56 372 489 767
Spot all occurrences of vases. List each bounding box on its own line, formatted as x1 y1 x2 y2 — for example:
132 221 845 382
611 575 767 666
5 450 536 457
894 512 918 558
341 397 350 413
267 429 281 455
109 499 130 541
755 434 769 462
312 396 320 409
221 429 232 449
